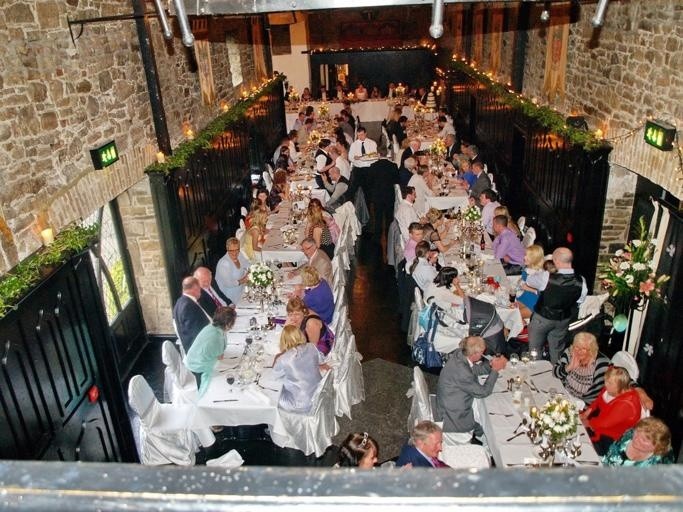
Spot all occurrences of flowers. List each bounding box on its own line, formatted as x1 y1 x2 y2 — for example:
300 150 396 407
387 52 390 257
597 213 671 316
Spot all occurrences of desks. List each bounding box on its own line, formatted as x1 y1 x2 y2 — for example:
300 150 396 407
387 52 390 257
283 97 416 134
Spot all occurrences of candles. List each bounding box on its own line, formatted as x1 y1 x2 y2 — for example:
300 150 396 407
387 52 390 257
40 227 54 248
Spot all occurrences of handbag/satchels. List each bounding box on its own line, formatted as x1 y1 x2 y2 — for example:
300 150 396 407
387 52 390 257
412 332 442 367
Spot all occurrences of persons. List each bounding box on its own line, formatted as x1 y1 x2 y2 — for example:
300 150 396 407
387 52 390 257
171 70 678 472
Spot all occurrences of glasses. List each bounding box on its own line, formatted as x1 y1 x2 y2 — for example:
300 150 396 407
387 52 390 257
573 346 590 353
228 249 240 252
301 244 312 252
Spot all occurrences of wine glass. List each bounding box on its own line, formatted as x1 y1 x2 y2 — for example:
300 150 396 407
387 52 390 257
507 346 540 418
225 257 284 397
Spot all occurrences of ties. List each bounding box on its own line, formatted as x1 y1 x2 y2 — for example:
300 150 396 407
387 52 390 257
432 457 449 468
362 142 365 156
209 288 222 308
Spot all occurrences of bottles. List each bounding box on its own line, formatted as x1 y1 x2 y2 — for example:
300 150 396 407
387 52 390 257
451 206 485 295
